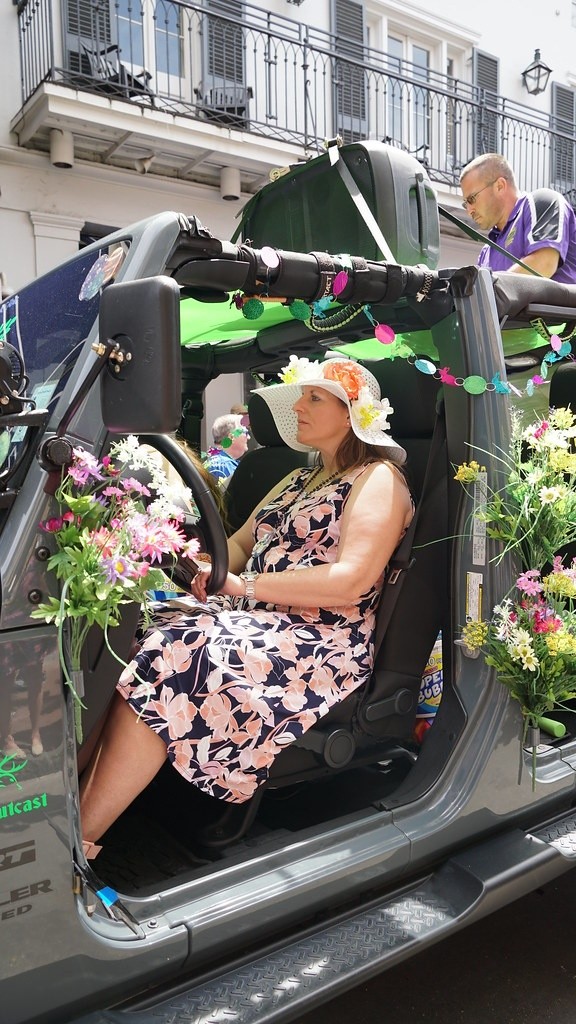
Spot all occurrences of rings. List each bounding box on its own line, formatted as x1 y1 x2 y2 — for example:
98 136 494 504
198 568 202 575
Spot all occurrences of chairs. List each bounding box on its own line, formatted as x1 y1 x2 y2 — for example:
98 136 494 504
161 354 457 855
452 155 474 185
381 136 429 175
79 38 159 106
194 86 253 131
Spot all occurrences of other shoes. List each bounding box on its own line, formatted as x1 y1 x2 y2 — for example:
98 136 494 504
31 737 43 756
3 747 26 759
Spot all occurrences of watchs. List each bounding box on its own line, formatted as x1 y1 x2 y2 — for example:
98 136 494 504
239 572 259 600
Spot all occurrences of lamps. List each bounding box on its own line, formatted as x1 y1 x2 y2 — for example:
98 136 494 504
520 48 553 95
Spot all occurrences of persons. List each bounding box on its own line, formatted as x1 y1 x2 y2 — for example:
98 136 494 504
201 405 258 488
458 153 576 285
79 355 414 863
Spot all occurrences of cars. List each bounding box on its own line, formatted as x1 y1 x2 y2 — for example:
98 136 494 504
0 133 576 1024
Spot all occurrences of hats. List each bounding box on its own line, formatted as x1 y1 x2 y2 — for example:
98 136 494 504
249 354 407 463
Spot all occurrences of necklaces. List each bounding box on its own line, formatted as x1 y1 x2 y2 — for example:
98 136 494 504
275 464 348 528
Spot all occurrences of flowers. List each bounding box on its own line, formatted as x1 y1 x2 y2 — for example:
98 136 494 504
31 433 216 746
456 402 575 584
277 352 394 436
455 547 576 793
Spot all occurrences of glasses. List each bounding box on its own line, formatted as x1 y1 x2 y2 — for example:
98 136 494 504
462 177 507 210
240 430 249 437
239 413 249 416
